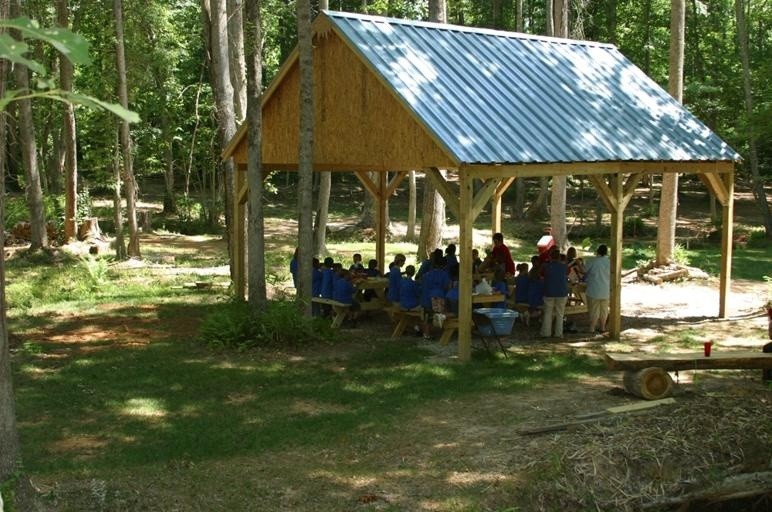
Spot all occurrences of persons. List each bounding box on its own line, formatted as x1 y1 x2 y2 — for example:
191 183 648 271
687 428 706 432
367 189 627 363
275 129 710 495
287 234 611 342
760 309 772 380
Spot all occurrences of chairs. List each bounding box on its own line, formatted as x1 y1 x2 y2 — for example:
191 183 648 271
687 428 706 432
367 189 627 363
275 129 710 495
473 311 510 361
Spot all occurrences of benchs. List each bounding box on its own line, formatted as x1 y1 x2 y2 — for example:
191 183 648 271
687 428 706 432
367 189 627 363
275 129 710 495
606 352 772 401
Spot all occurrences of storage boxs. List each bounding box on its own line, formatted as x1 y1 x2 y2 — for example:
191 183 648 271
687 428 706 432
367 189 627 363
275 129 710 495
472 307 519 337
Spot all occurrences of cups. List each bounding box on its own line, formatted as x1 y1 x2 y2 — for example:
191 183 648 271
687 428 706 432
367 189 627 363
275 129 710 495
705 342 712 357
